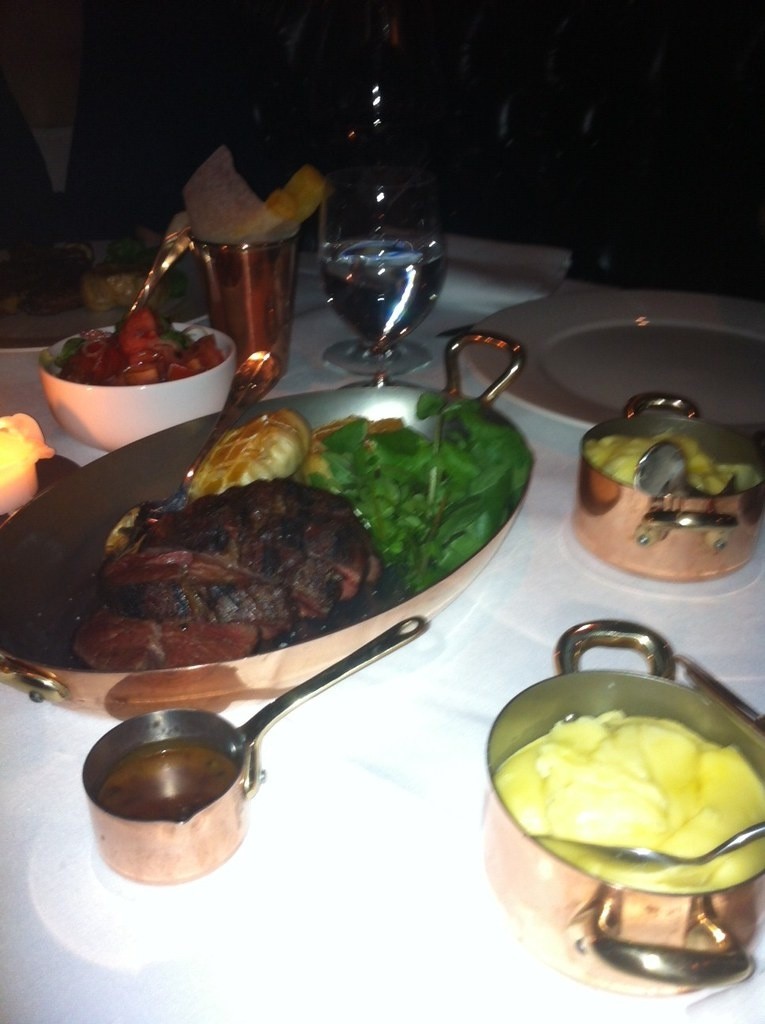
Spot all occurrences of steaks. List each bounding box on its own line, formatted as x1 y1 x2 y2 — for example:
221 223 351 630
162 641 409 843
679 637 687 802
69 483 382 674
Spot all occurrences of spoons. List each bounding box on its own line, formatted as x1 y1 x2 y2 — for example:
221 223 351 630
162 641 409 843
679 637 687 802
632 440 702 502
536 820 764 871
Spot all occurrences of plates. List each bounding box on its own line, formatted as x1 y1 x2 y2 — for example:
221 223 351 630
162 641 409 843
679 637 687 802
460 283 764 445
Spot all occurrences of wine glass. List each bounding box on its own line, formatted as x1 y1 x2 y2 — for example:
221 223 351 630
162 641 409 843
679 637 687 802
315 56 442 390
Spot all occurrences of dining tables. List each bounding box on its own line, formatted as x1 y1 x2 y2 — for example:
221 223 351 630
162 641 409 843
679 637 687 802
2 272 764 1024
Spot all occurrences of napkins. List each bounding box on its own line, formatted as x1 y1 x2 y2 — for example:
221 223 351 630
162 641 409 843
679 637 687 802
367 224 574 318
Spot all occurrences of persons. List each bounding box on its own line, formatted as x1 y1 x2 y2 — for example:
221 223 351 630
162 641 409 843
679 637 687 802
1 1 204 252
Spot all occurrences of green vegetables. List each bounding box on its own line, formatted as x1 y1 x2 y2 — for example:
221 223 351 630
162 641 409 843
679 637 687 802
317 394 528 592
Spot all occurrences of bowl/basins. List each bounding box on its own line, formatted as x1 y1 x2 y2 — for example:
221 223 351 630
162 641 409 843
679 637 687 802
42 320 228 451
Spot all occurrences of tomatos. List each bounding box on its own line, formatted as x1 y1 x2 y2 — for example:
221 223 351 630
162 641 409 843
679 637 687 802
65 307 222 384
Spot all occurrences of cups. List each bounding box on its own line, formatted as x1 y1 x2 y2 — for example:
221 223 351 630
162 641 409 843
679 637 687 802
187 232 301 389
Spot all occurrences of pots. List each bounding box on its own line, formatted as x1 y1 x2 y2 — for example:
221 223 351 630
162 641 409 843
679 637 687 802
6 328 536 712
480 620 763 1001
80 616 431 885
570 390 763 587
